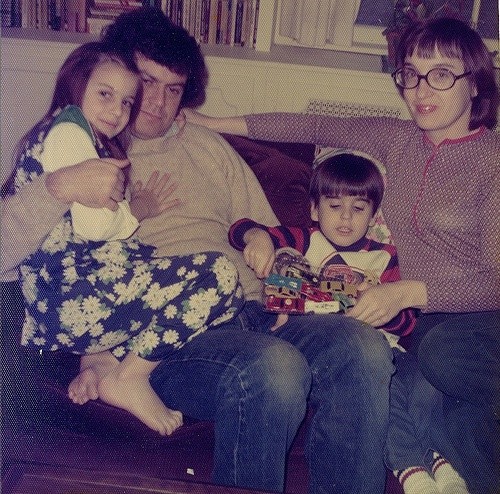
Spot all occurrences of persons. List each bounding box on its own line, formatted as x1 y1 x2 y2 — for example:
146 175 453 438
228 154 470 494
15 41 247 435
1 6 396 493
173 17 500 494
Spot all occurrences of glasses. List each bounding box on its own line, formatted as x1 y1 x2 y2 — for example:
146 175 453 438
391 66 472 91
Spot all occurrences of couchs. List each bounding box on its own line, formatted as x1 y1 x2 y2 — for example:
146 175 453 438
1 132 314 480
